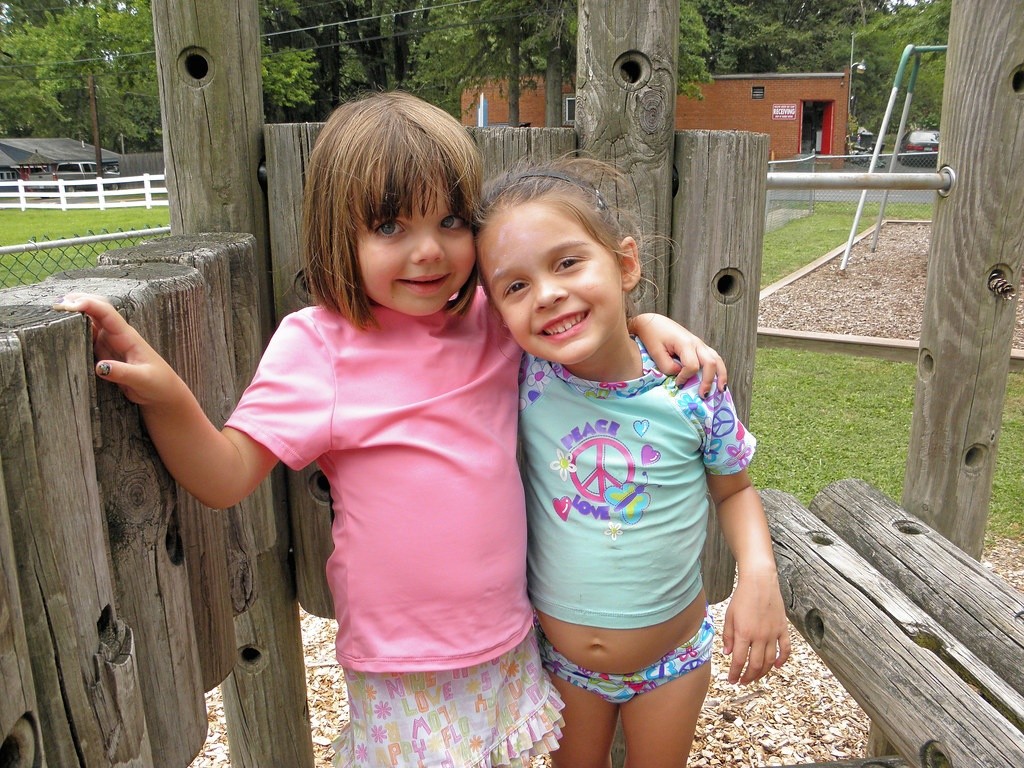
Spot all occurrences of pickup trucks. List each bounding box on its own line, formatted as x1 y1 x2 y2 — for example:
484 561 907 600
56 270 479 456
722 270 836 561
27 162 120 199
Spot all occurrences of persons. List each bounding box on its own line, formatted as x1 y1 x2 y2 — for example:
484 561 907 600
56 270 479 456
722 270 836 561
55 93 728 768
473 158 792 768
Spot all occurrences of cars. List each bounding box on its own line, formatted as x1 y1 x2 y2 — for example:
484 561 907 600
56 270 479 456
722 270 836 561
897 130 940 165
846 127 873 142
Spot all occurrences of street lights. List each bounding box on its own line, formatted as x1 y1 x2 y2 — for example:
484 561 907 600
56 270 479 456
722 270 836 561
847 32 867 112
